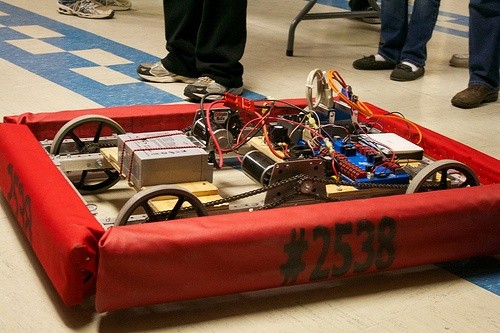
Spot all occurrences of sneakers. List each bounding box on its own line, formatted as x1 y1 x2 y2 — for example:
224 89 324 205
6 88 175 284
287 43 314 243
96 0 132 11
183 76 244 102
135 60 199 84
56 0 115 19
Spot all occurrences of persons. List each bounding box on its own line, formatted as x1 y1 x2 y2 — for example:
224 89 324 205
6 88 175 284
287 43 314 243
352 0 440 81
57 0 131 19
348 0 381 24
137 0 249 101
448 0 500 109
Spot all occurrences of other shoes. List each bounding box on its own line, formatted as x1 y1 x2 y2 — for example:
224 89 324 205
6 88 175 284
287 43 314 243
390 61 425 82
357 7 381 23
352 54 396 70
451 83 498 109
